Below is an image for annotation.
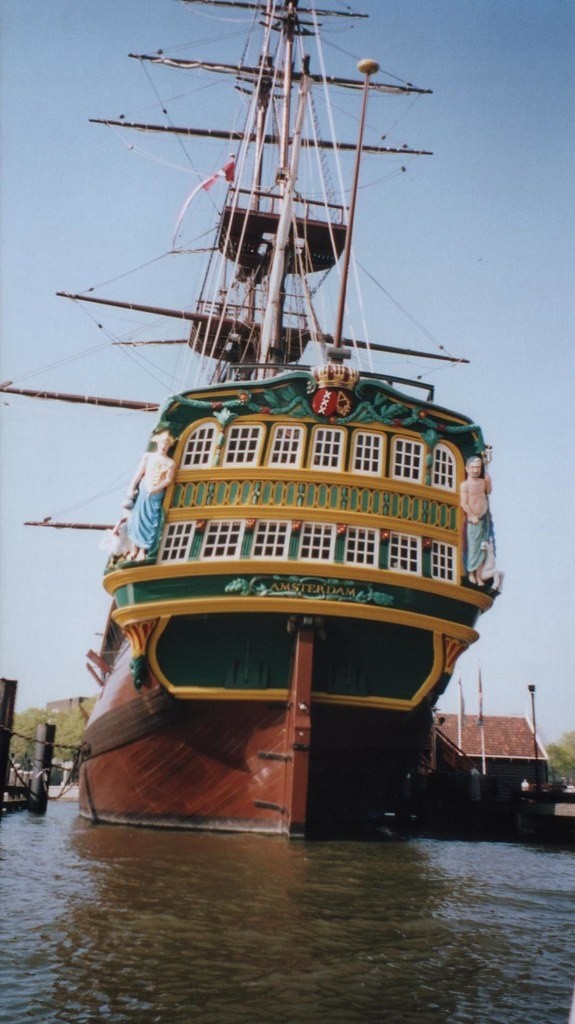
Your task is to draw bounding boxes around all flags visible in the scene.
[474,671,484,726]
[457,678,466,730]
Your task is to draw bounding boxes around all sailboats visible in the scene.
[0,0,504,840]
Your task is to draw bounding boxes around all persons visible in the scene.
[460,454,493,584]
[123,428,176,564]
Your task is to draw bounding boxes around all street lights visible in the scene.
[527,684,542,792]
